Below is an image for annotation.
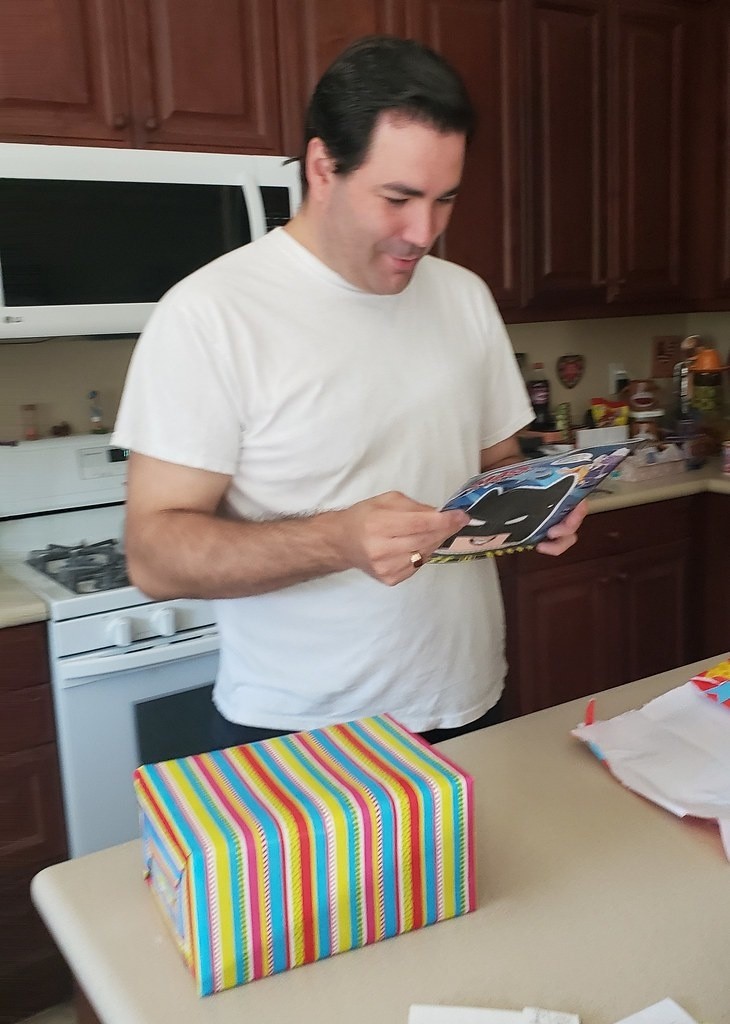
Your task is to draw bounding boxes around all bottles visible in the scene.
[527,363,549,432]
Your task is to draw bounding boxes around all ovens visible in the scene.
[52,626,224,856]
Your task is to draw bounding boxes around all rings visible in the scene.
[410,552,422,567]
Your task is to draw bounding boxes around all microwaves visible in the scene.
[1,143,302,339]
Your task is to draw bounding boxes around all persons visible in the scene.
[108,36,589,746]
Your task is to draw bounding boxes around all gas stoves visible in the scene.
[0,429,217,662]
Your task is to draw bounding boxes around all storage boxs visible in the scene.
[127,710,477,994]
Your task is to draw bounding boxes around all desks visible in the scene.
[28,649,730,1024]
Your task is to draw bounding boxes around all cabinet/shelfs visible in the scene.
[0,0,730,325]
[0,566,82,1024]
[494,458,730,722]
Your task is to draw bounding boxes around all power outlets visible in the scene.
[609,363,625,393]
